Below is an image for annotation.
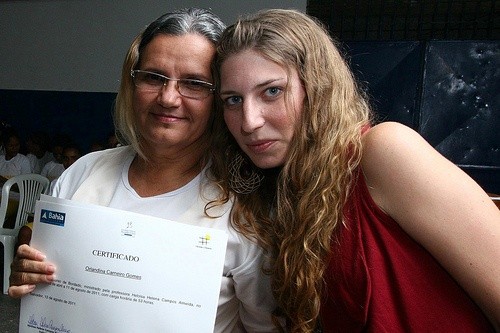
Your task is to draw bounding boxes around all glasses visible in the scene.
[130,68,216,98]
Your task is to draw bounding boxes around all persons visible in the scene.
[0,121,121,188]
[6,8,283,333]
[196,6,500,333]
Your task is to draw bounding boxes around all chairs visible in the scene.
[0,174,54,295]
